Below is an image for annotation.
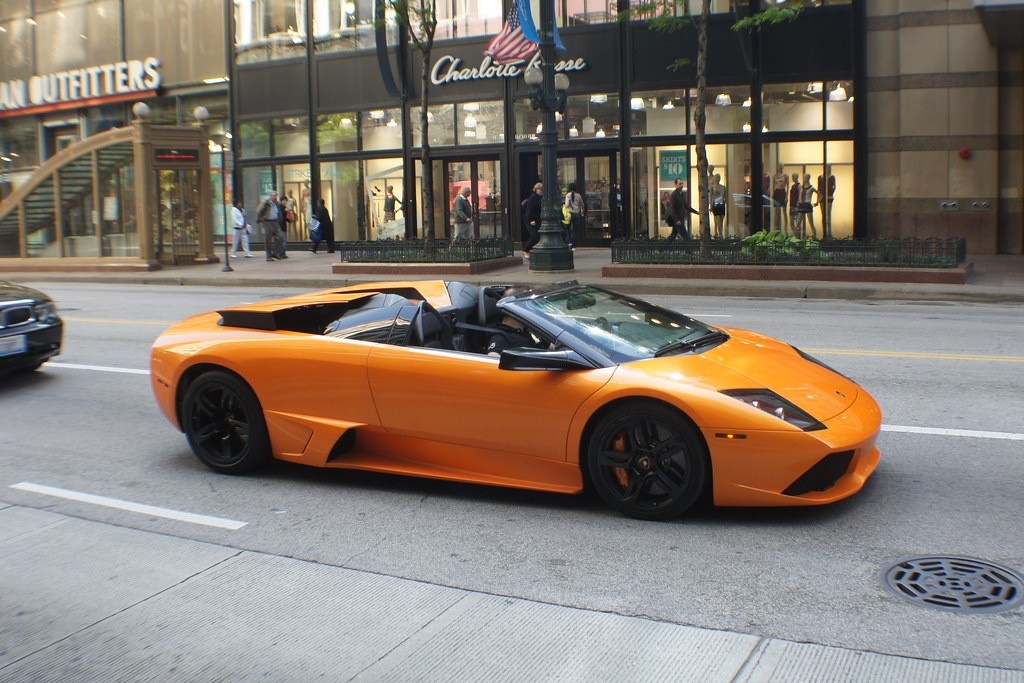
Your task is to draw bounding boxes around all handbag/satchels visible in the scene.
[561,205,571,224]
[246,222,252,233]
[308,217,320,233]
[665,207,673,226]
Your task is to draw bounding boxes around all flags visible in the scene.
[482,0,541,67]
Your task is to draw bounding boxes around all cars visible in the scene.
[0,280,63,378]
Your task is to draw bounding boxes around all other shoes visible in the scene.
[244,253,252,257]
[230,254,236,258]
[568,242,576,251]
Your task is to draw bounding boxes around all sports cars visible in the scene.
[149,278,888,524]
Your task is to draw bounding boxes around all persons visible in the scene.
[789,172,803,236]
[229,197,254,259]
[661,192,670,221]
[449,187,477,256]
[285,189,298,241]
[522,183,544,258]
[308,199,336,253]
[256,192,290,262]
[564,183,585,251]
[300,180,312,241]
[818,162,836,238]
[486,283,556,359]
[706,165,728,241]
[382,185,402,242]
[666,178,700,241]
[772,162,789,238]
[495,192,501,211]
[795,172,823,240]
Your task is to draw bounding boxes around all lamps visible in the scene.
[582,95,596,135]
[0,141,21,162]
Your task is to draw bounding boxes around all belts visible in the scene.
[265,219,276,221]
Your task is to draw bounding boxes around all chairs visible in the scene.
[478,286,503,328]
[407,300,466,352]
